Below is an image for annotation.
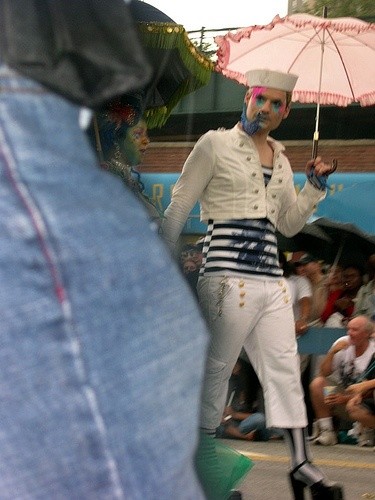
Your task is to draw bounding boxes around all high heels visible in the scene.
[288,459,343,500]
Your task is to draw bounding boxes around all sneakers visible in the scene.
[314,431,338,445]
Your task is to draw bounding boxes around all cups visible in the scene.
[323,386,337,401]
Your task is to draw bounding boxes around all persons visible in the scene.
[177,245,375,445]
[159,69,343,500]
[96,105,161,227]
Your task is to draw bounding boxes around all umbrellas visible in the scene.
[274,223,333,252]
[212,6,375,177]
[311,217,375,261]
[122,0,212,129]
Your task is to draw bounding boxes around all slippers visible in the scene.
[251,426,269,442]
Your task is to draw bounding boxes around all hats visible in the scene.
[245,69,299,92]
